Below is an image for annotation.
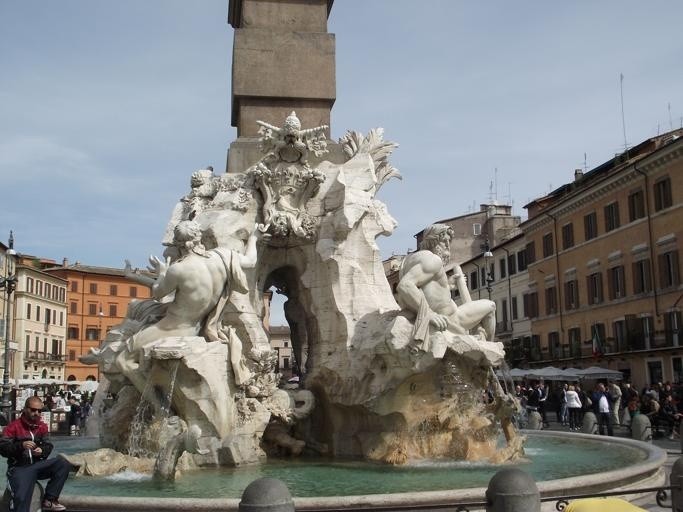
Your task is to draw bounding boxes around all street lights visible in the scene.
[96,304,104,383]
[483,237,492,300]
[0,228,18,427]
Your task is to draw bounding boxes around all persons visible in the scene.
[0,370,99,447]
[393,223,498,344]
[77,219,271,423]
[496,371,683,447]
[0,394,71,511]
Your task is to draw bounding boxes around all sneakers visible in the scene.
[42,499,67,511]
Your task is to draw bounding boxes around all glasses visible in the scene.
[27,407,42,413]
